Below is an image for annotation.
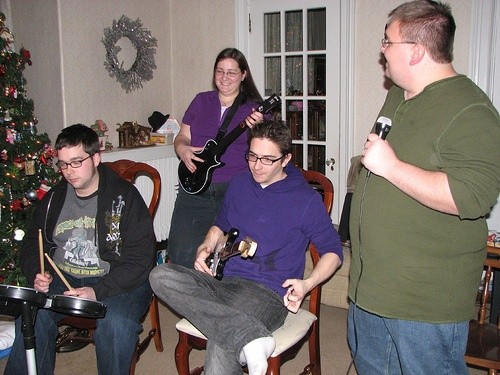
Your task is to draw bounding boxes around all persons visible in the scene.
[3,123,158,375]
[347,0,500,375]
[166,48,266,267]
[147,119,344,375]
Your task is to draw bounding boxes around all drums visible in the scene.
[0,284,47,310]
[49,294,107,319]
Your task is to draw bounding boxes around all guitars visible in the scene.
[177,93,281,195]
[205,228,258,281]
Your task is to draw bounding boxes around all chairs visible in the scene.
[172,170,334,375]
[40,160,163,375]
[463,256,500,375]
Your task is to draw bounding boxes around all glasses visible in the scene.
[56,156,90,169]
[245,150,282,165]
[380,37,414,48]
[215,68,242,77]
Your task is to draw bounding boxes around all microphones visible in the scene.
[367,116,392,177]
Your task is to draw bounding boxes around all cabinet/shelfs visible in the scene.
[100,142,180,265]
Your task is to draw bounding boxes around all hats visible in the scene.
[148,110,170,134]
